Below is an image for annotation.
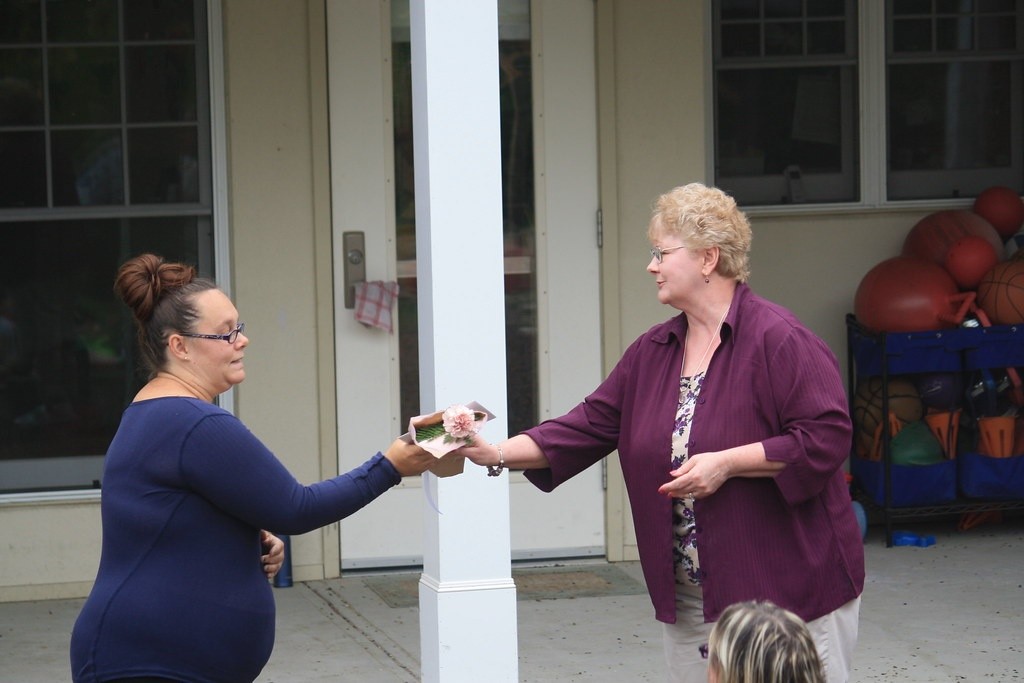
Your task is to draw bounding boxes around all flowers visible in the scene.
[408,405,487,478]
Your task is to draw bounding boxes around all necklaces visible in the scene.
[677,297,730,404]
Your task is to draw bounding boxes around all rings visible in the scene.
[688,491,697,504]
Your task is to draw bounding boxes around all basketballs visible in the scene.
[854,376,922,439]
[978,260,1024,325]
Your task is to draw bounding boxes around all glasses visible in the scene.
[696,643,709,659]
[649,246,687,263]
[165,322,246,344]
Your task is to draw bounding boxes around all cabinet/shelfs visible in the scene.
[846,313,1024,549]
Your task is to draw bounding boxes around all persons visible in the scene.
[68,253,444,683]
[705,596,829,683]
[453,182,866,683]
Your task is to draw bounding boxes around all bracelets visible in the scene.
[486,438,508,480]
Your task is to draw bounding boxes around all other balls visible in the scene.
[946,236,997,287]
[1004,230,1024,260]
[888,421,944,465]
[917,372,975,411]
[974,186,1024,236]
[852,256,959,330]
[903,210,1005,290]
